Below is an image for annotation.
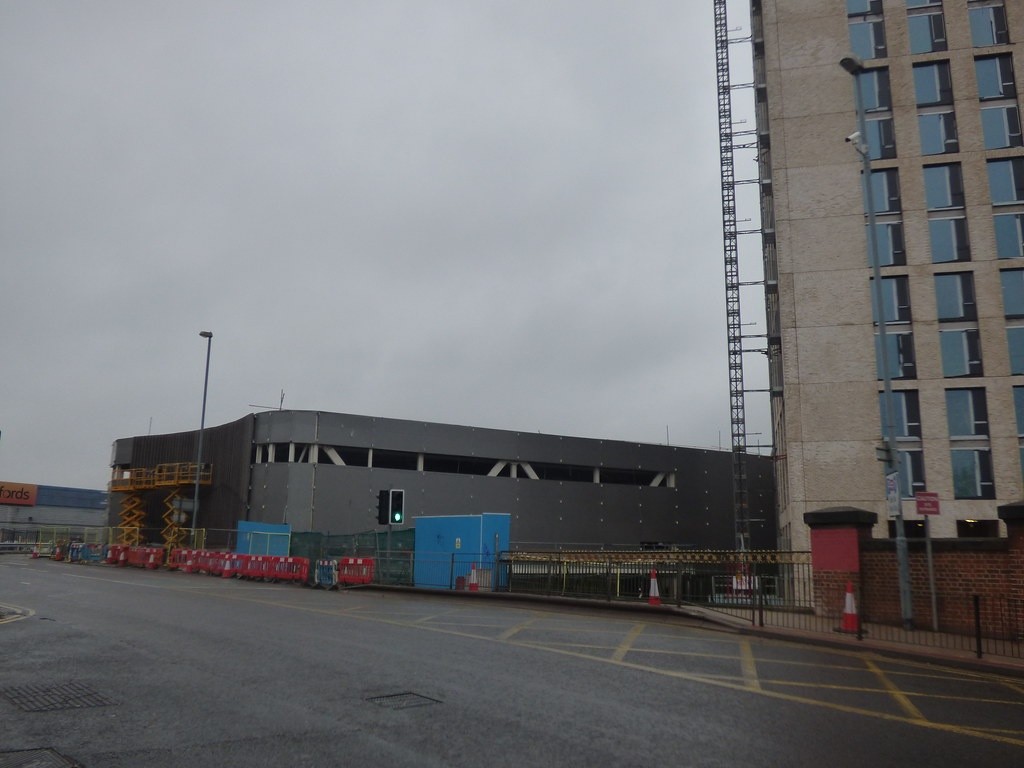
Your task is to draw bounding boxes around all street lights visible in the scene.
[189,331,213,545]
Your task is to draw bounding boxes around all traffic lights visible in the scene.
[375,491,389,524]
[388,488,404,525]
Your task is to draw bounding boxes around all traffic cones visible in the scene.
[186,555,193,573]
[222,557,231,576]
[829,578,870,634]
[468,563,479,593]
[118,551,128,568]
[106,548,114,565]
[146,549,157,570]
[55,546,62,562]
[648,568,662,605]
[29,545,39,559]
